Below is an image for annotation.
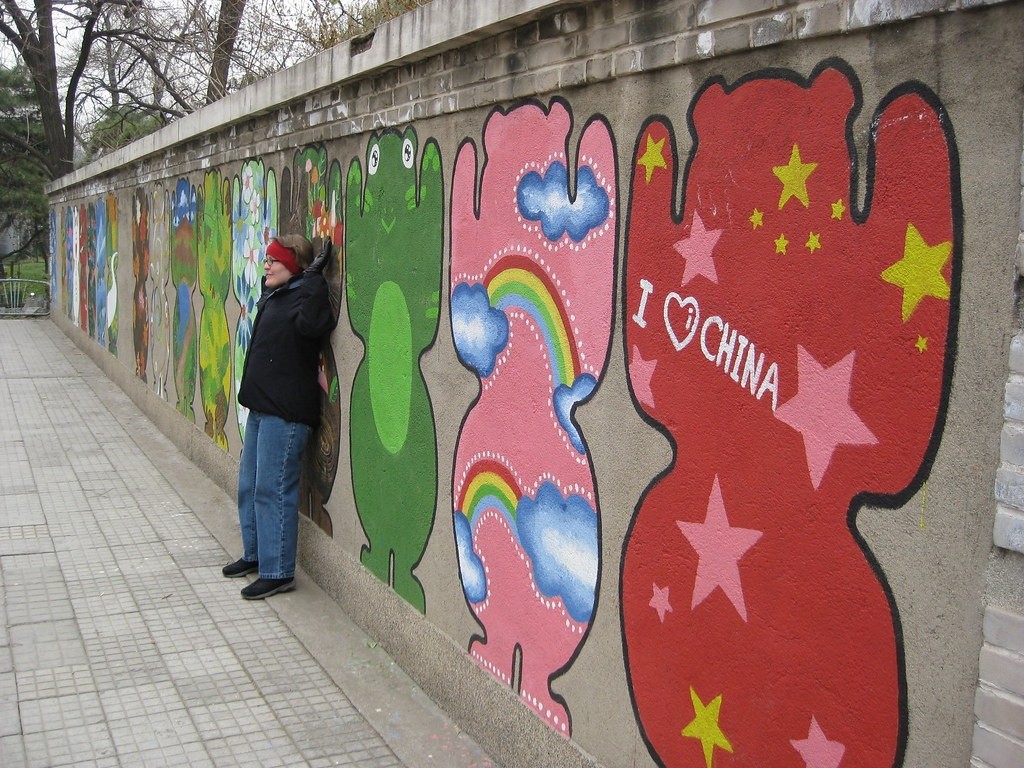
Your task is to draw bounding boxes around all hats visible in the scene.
[266,240,302,274]
[270,235,314,268]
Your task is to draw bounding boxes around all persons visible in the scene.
[222,234,332,600]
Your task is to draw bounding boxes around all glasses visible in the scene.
[263,257,280,265]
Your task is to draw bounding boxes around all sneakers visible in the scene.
[241,576,296,600]
[222,557,258,577]
